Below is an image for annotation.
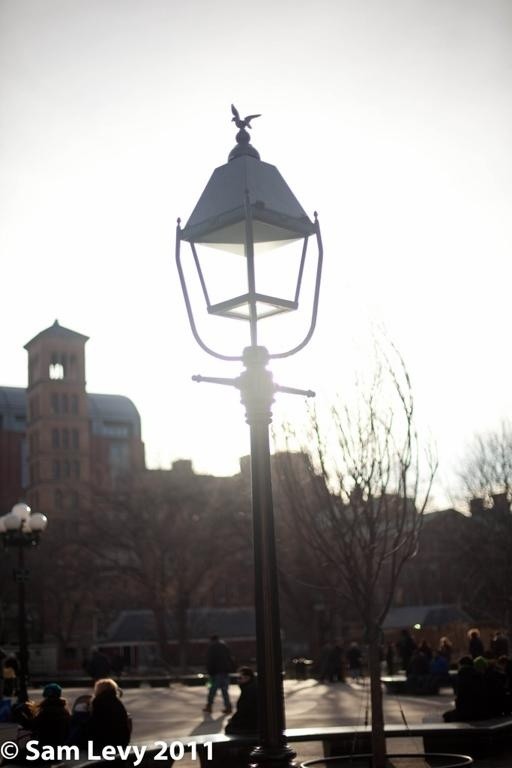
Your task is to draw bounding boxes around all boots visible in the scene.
[203,703,211,712]
[223,705,231,713]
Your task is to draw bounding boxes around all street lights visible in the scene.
[176,104,323,768]
[0,501,48,672]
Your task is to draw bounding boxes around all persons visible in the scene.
[0,646,132,765]
[320,629,512,722]
[226,666,258,734]
[204,634,236,713]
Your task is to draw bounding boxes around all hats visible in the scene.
[42,683,62,697]
